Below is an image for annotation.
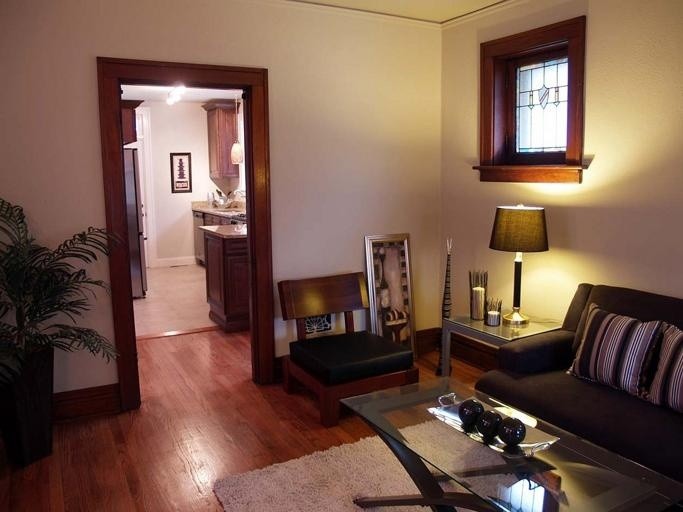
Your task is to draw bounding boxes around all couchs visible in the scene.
[473,283,682,483]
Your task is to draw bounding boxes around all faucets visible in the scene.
[233,189,245,197]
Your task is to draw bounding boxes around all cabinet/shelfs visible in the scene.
[200,98,239,179]
[192,206,250,332]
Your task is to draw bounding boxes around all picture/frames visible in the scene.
[170,152,192,193]
[363,232,417,361]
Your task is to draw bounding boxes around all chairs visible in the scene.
[276,271,419,428]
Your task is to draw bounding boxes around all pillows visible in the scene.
[564,302,683,409]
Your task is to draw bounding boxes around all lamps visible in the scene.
[487,205,549,327]
[230,98,242,165]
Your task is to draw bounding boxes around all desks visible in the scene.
[440,313,562,390]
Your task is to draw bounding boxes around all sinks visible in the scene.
[215,208,243,213]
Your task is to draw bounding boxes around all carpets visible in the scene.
[211,420,518,512]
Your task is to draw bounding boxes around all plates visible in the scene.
[426,392,559,458]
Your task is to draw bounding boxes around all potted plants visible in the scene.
[0,198,125,471]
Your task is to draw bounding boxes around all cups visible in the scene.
[207,192,214,209]
[219,197,224,211]
